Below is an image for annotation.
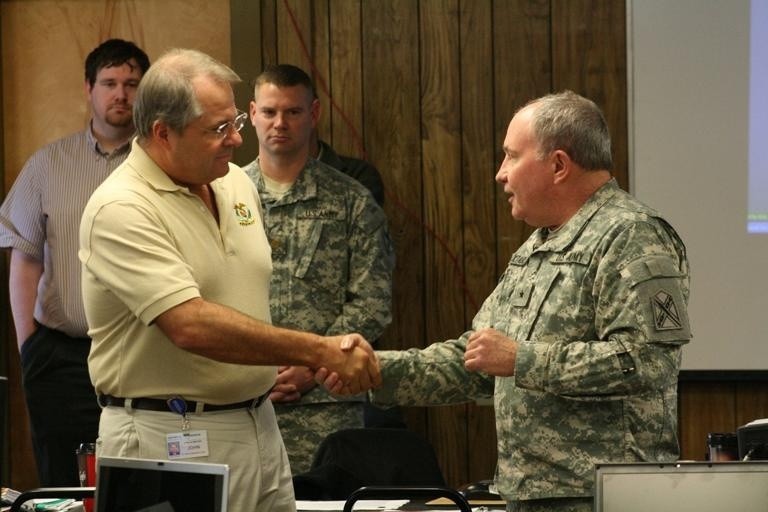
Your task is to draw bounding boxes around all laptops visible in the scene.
[96,456,230,512]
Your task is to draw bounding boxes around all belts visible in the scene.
[101,389,275,413]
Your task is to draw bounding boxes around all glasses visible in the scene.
[155,108,248,139]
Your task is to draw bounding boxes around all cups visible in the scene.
[708,433,740,461]
[76,443,96,512]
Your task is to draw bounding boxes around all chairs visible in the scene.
[292,428,446,499]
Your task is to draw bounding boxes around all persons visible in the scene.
[77,48,384,512]
[312,90,694,512]
[236,63,396,478]
[307,123,384,210]
[0,37,153,489]
